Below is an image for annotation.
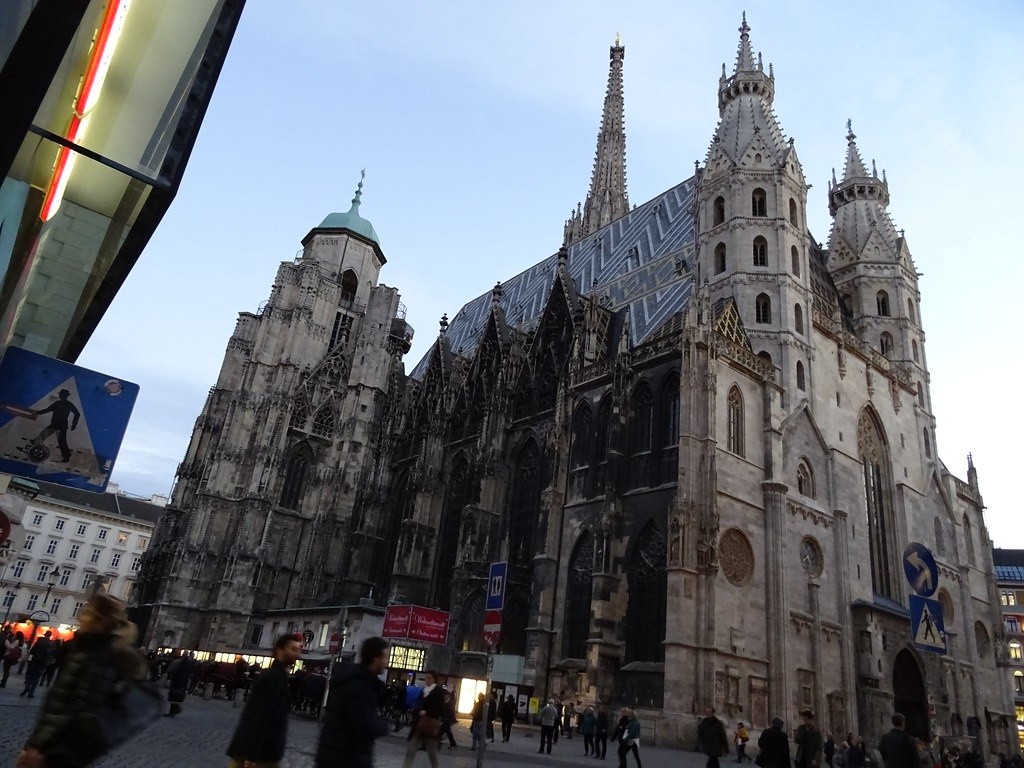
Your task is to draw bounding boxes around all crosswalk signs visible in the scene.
[909,594,948,654]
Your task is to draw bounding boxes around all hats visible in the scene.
[76,595,137,638]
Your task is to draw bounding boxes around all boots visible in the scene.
[163,703,177,718]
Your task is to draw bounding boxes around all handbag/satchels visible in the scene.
[68,673,167,764]
[415,715,443,737]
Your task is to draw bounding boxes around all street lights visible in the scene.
[17,564,61,674]
[1,581,22,632]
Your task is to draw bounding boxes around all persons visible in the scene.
[17,593,163,768]
[578,703,610,760]
[225,634,300,768]
[821,713,1024,768]
[390,670,457,768]
[793,710,823,768]
[755,717,791,768]
[611,707,643,768]
[469,691,518,751]
[733,722,753,764]
[1,625,228,719]
[537,697,575,755]
[696,707,730,768]
[314,637,396,768]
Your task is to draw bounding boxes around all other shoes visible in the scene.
[468,746,477,751]
[590,748,594,756]
[594,755,600,759]
[536,749,543,753]
[489,738,494,744]
[448,744,457,749]
[501,735,509,743]
[600,755,606,761]
[583,752,588,756]
[20,688,34,698]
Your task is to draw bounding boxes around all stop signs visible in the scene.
[483,611,501,649]
[329,633,340,654]
[295,632,304,651]
